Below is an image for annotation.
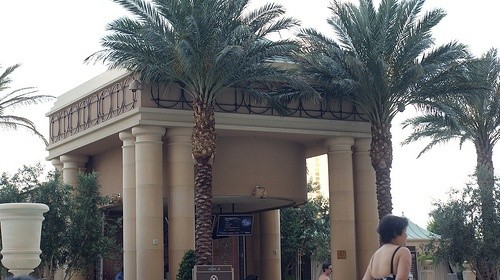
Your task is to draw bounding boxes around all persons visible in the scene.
[361,215,413,280]
[319,263,333,279]
[115,268,123,280]
